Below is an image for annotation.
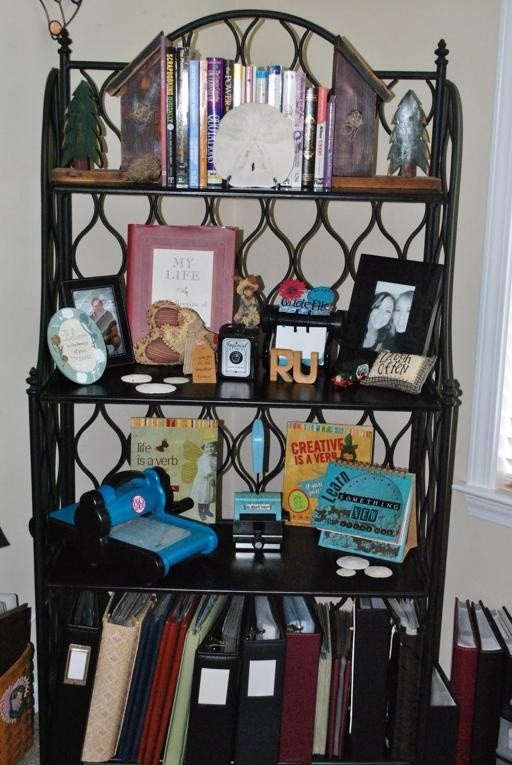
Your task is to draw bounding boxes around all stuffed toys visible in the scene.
[230,273,263,328]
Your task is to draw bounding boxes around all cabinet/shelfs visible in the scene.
[26,3,467,764]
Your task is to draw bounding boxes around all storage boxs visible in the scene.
[1,632,40,765]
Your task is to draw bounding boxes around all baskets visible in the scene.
[0,643,34,765]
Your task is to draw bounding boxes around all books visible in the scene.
[282,419,376,528]
[161,29,337,194]
[1,593,511,765]
[312,458,418,566]
[130,416,220,527]
[127,223,240,366]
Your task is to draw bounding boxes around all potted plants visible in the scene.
[279,278,307,303]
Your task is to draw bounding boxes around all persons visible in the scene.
[358,291,394,353]
[87,298,120,355]
[388,290,413,353]
[190,441,218,521]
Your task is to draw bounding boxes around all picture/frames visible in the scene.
[57,273,133,368]
[128,221,238,344]
[337,248,443,377]
